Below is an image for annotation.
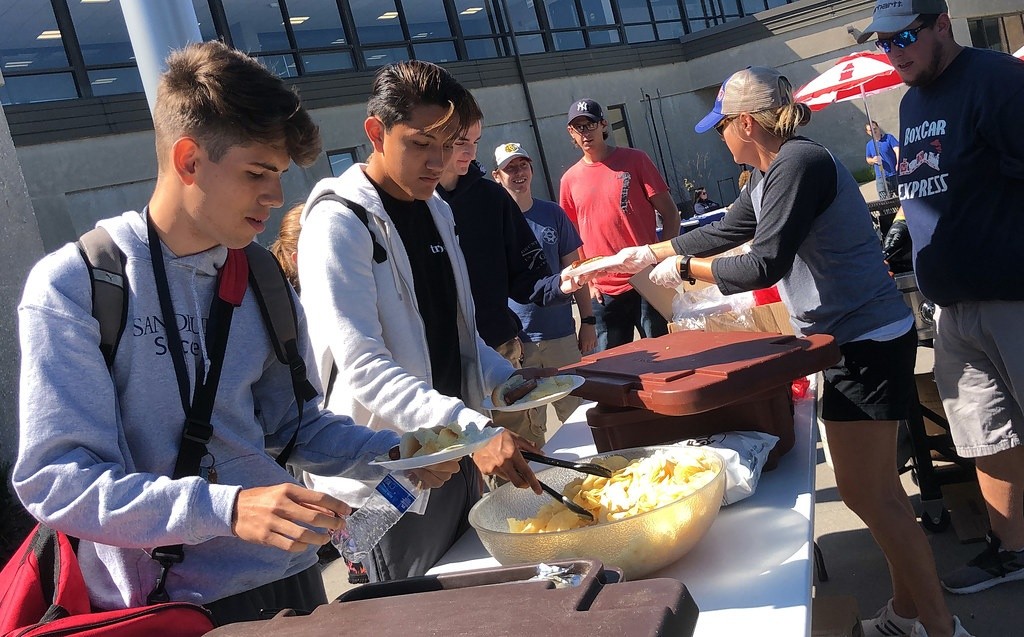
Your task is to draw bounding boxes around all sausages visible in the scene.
[572,255,604,268]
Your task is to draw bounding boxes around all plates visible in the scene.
[368,427,506,472]
[478,374,585,412]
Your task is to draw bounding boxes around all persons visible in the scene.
[865,121,900,199]
[12,40,462,629]
[858,0,1024,594]
[296,61,558,583]
[693,187,720,215]
[272,89,598,370]
[605,65,971,637]
[560,99,681,352]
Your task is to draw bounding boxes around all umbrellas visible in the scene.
[794,49,906,198]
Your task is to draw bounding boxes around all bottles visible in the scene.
[331,471,424,564]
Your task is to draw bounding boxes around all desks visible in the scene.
[656,207,755,258]
[422,373,820,637]
[908,343,977,534]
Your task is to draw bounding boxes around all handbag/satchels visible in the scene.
[0,520,216,637]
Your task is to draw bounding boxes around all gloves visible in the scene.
[884,220,912,259]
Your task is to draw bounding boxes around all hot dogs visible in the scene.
[491,375,539,407]
[375,425,446,461]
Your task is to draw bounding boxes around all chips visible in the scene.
[529,375,574,401]
[412,419,467,456]
[505,448,720,537]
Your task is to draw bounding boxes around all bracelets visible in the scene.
[680,255,695,285]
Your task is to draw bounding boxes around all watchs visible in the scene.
[581,316,596,325]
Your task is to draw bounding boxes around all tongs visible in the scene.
[519,450,612,523]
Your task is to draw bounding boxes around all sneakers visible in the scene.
[941,531,1024,595]
[860,597,972,637]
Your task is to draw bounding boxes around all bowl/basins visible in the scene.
[468,444,727,581]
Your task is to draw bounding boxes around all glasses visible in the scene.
[700,192,708,195]
[714,113,743,137]
[572,122,599,133]
[874,20,929,53]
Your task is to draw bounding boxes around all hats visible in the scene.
[492,142,533,170]
[857,0,948,45]
[694,65,792,135]
[567,99,604,123]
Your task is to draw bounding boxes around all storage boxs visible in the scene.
[197,561,700,637]
[555,330,843,460]
